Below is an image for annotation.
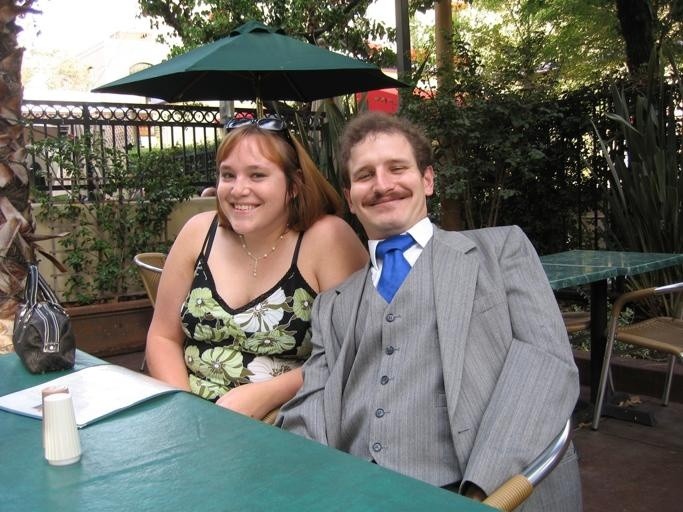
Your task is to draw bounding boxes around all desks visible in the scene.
[0,347,509,512]
[539,249,683,430]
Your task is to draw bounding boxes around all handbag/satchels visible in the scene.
[12,265,75,375]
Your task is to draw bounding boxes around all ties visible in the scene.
[376,232,417,304]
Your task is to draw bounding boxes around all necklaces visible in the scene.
[236,223,290,276]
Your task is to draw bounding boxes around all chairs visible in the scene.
[591,281,683,430]
[562,312,615,397]
[133,252,166,372]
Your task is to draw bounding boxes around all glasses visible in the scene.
[226,118,287,133]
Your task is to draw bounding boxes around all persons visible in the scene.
[142,112,373,447]
[271,108,585,512]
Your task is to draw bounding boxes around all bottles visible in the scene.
[42,385,84,466]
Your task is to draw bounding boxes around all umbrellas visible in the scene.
[88,18,414,120]
